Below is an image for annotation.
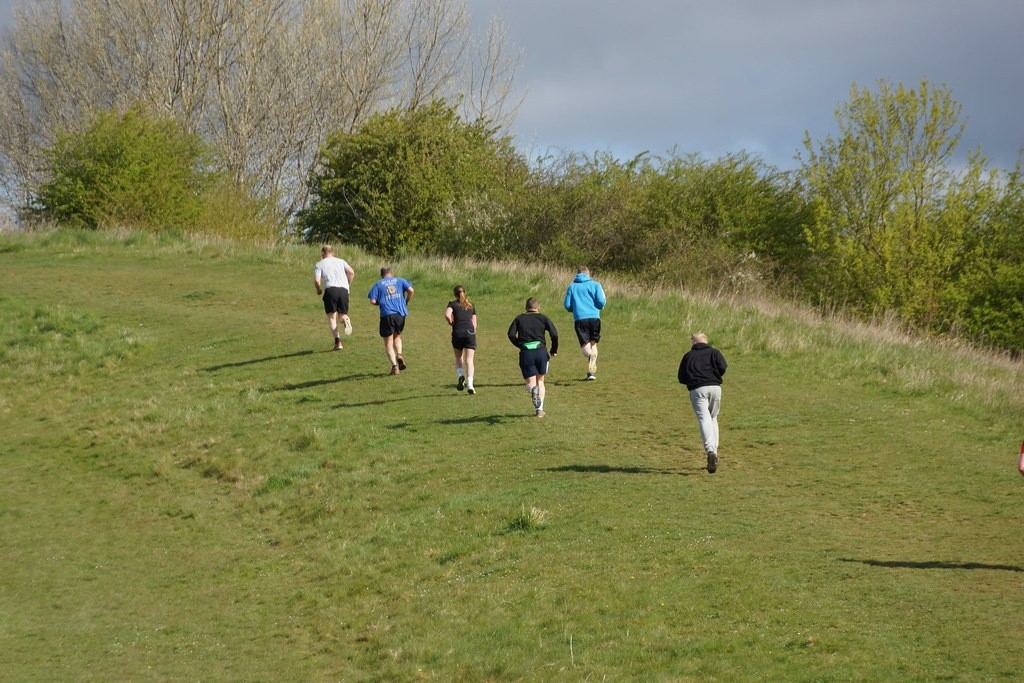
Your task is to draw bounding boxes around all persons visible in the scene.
[444,285,477,394]
[314,245,355,350]
[678,333,728,473]
[564,265,606,380]
[508,297,558,418]
[368,267,414,375]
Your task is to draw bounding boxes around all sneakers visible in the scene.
[586,353,598,380]
[457,375,465,391]
[344,317,353,336]
[707,451,718,473]
[396,355,406,370]
[536,410,545,418]
[333,341,344,350]
[391,366,399,375]
[467,387,476,394]
[531,386,541,408]
